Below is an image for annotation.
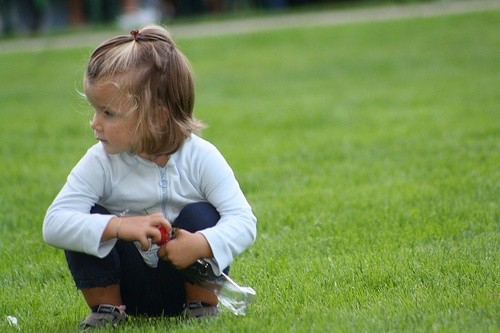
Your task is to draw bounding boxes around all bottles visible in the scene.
[155,226,256,318]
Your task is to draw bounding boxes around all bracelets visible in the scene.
[116,217,121,239]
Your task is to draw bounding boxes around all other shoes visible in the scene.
[186,301,218,317]
[79,304,127,330]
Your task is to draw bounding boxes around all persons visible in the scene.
[41,24,258,331]
